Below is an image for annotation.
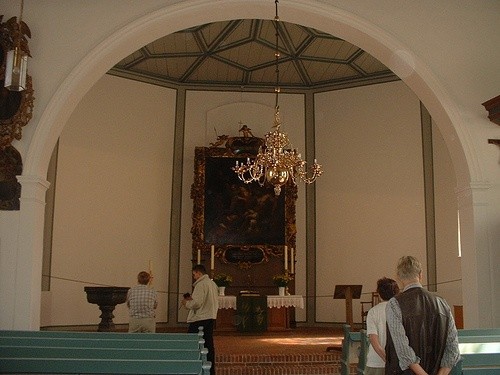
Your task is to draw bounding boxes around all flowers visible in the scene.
[211,272,233,289]
[271,269,295,287]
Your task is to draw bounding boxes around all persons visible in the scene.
[385,255,459,375]
[182,264,219,375]
[126,271,158,333]
[364,277,401,375]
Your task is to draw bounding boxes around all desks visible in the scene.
[213,296,304,337]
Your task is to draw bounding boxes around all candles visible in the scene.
[197,249,201,265]
[210,245,214,270]
[284,245,288,269]
[290,248,295,274]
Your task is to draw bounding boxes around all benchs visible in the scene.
[0,326,212,375]
[340,323,500,375]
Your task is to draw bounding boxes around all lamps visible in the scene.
[3,0,28,92]
[234,0,325,195]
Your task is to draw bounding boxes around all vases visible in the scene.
[219,287,226,297]
[278,287,286,297]
